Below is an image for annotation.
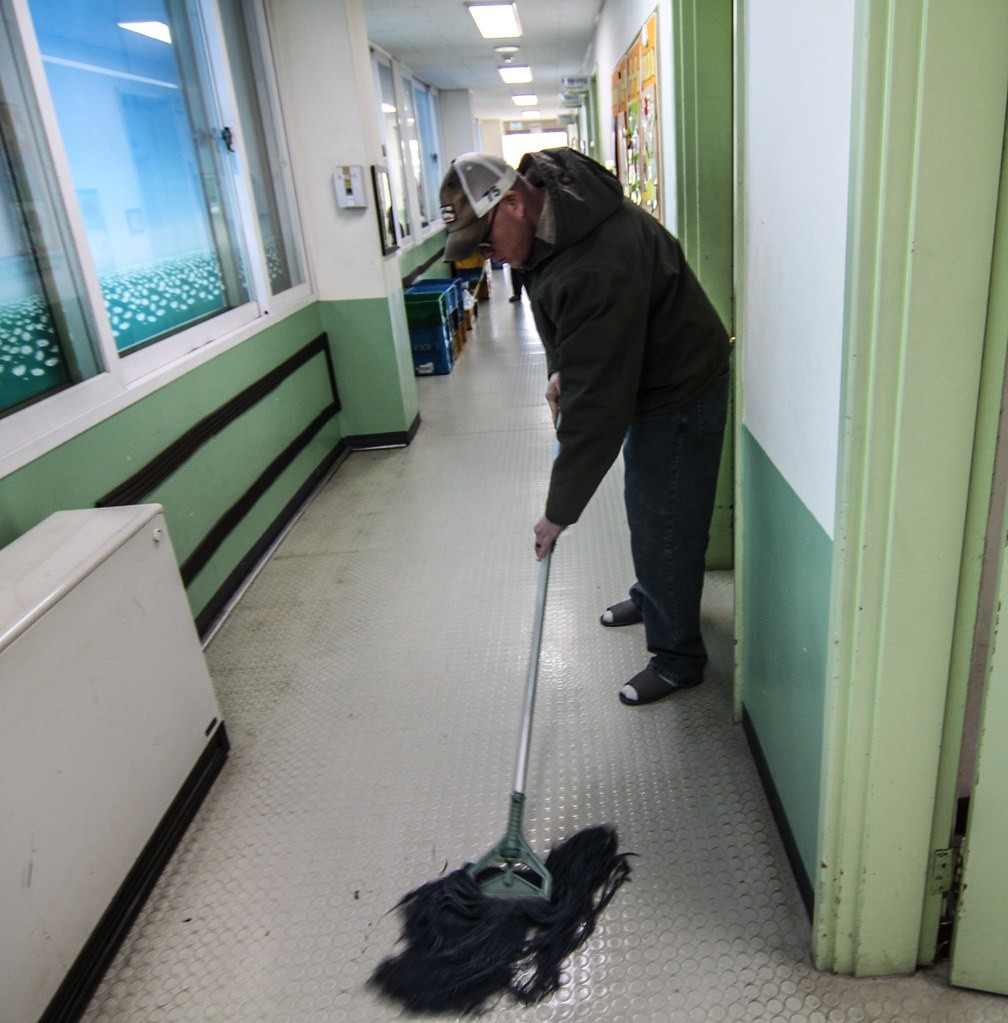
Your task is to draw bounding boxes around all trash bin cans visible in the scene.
[404,278,468,375]
[454,251,492,299]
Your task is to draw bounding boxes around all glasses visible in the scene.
[478,205,499,252]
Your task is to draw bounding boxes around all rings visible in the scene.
[535,542,541,548]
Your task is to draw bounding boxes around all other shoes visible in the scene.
[509,295,520,302]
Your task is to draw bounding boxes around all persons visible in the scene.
[437,144,729,706]
[507,267,529,302]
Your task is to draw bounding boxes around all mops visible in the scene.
[362,393,641,1022]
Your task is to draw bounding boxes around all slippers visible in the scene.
[619,667,704,705]
[600,598,643,627]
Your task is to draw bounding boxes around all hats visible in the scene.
[440,150,517,263]
